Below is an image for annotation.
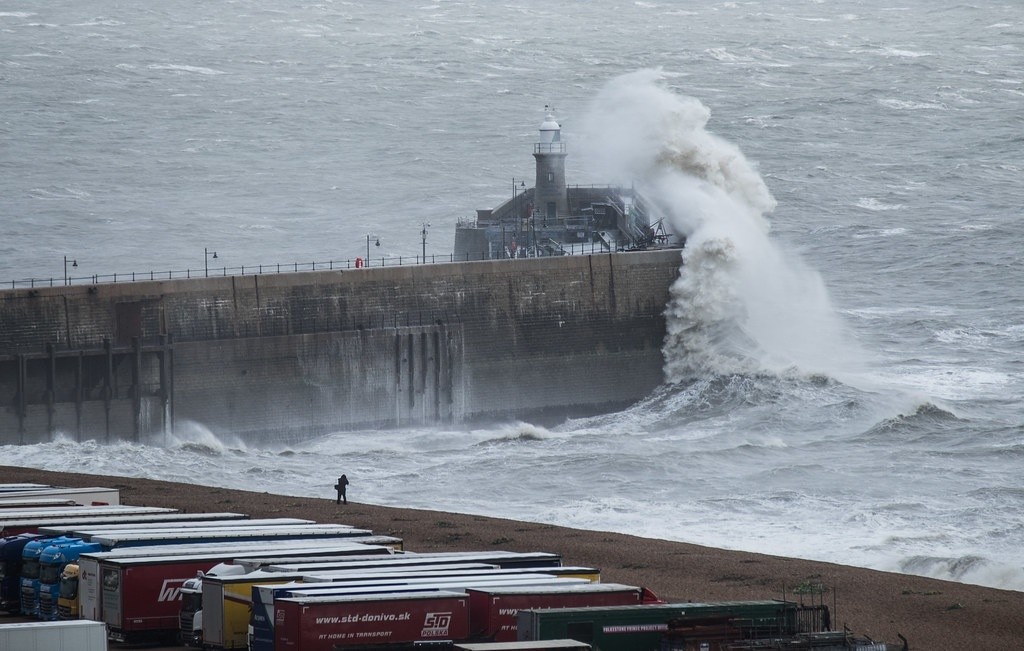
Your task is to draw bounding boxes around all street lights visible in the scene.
[205,248,219,278]
[421,221,431,264]
[64,256,78,287]
[502,178,541,260]
[366,234,380,268]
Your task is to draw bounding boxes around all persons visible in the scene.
[336,474,349,505]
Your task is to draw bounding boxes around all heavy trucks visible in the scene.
[0,480,922,650]
